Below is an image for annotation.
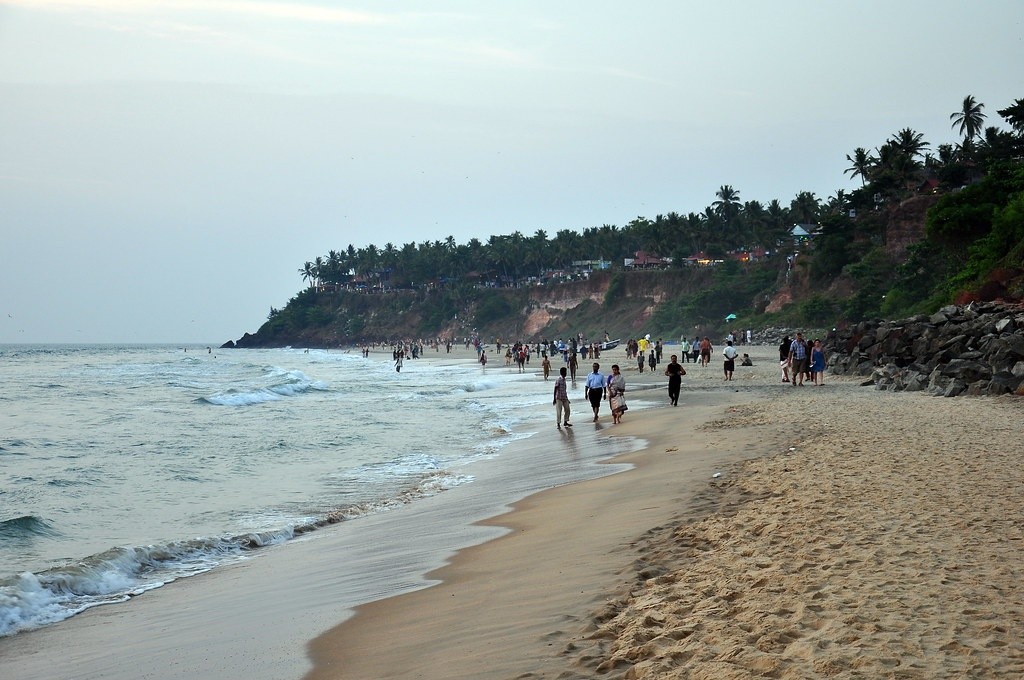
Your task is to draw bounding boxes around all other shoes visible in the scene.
[793,378,796,386]
[805,379,810,381]
[799,383,804,386]
[785,378,790,382]
[782,379,785,382]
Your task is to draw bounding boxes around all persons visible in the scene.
[353,331,826,429]
[664,355,686,406]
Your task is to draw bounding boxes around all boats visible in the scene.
[559,338,621,354]
